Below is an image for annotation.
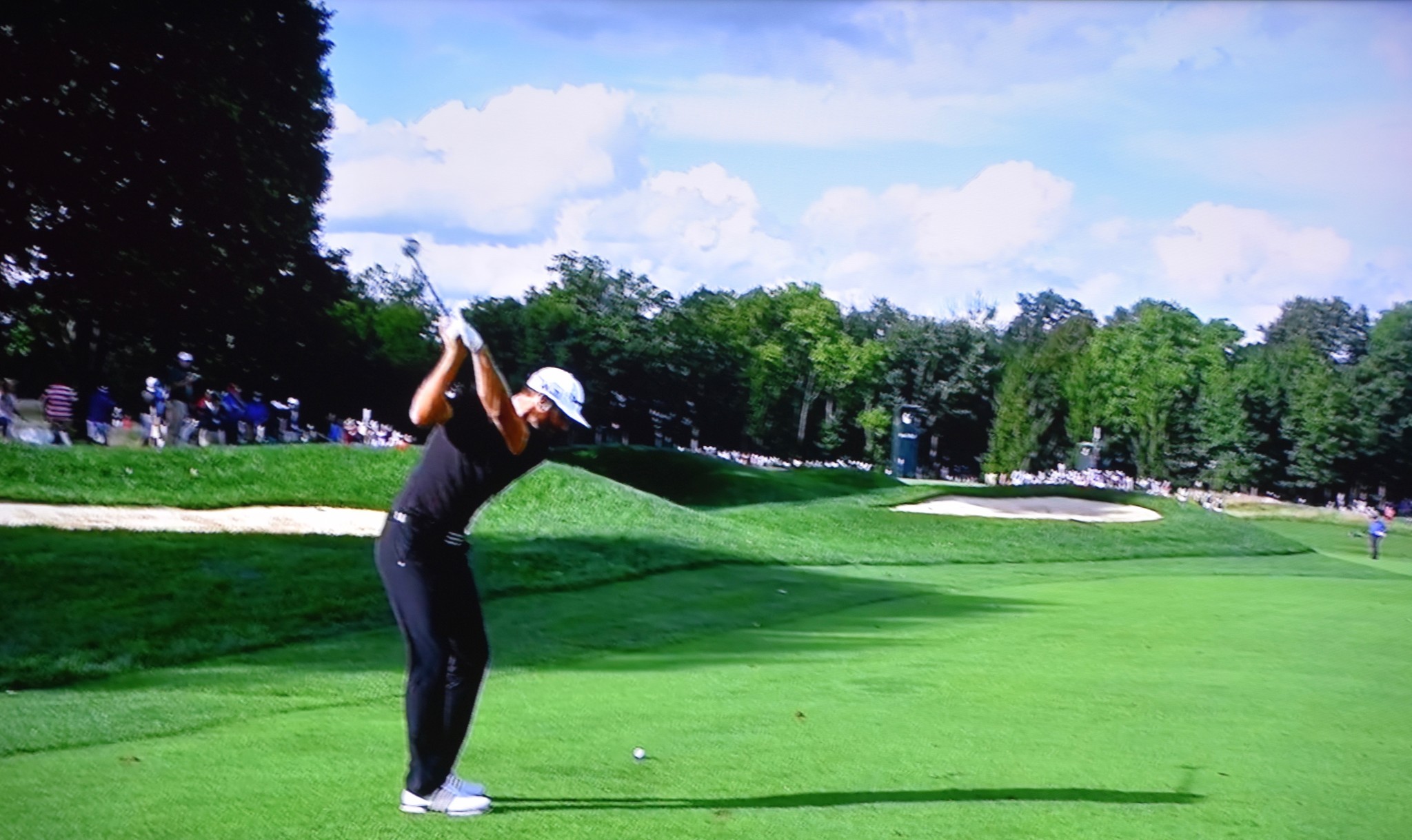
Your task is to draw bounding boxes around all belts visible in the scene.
[392,509,467,546]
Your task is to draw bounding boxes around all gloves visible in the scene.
[444,313,484,352]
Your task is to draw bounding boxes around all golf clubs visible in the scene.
[399,237,450,317]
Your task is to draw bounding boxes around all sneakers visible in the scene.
[442,774,487,796]
[398,780,491,817]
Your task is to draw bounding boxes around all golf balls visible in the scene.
[632,746,646,759]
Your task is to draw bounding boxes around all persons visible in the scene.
[375,308,592,814]
[1368,510,1387,559]
[0,376,410,447]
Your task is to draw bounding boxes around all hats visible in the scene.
[527,366,591,430]
